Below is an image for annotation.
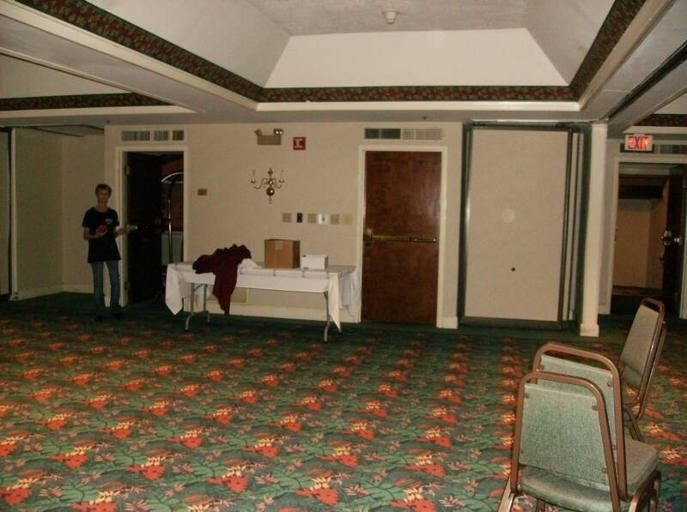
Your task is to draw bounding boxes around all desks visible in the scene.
[168,260,356,344]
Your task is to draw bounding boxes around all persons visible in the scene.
[81,184,128,314]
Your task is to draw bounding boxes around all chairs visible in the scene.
[510,337,663,512]
[606,295,674,442]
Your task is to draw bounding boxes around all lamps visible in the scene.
[248,165,288,204]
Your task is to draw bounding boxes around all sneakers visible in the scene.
[95,316,103,323]
[112,311,123,320]
